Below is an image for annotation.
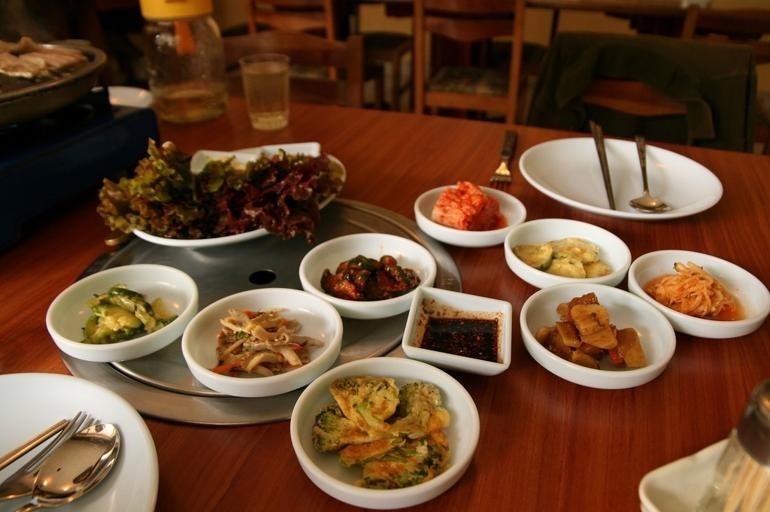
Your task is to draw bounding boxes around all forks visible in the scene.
[487,128,520,192]
[0,408,95,502]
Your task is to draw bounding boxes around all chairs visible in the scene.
[405,1,526,126]
[526,29,762,150]
[236,0,387,109]
[214,31,368,115]
[677,1,770,72]
[345,0,415,113]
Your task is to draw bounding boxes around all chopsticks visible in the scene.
[590,122,616,212]
[0,418,68,473]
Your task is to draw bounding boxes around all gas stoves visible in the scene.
[0,85,161,254]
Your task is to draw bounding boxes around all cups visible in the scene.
[238,51,291,130]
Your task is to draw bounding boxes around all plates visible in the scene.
[299,232,440,321]
[401,285,513,377]
[183,287,344,397]
[503,218,632,290]
[125,153,348,248]
[47,264,199,363]
[519,283,676,390]
[108,82,156,110]
[637,436,728,512]
[287,357,481,511]
[514,135,725,220]
[628,250,770,340]
[0,369,159,512]
[414,184,527,248]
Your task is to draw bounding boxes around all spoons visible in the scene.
[15,414,121,512]
[625,130,667,213]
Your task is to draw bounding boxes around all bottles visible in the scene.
[697,380,770,512]
[139,1,230,124]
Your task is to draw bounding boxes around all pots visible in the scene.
[1,39,109,125]
